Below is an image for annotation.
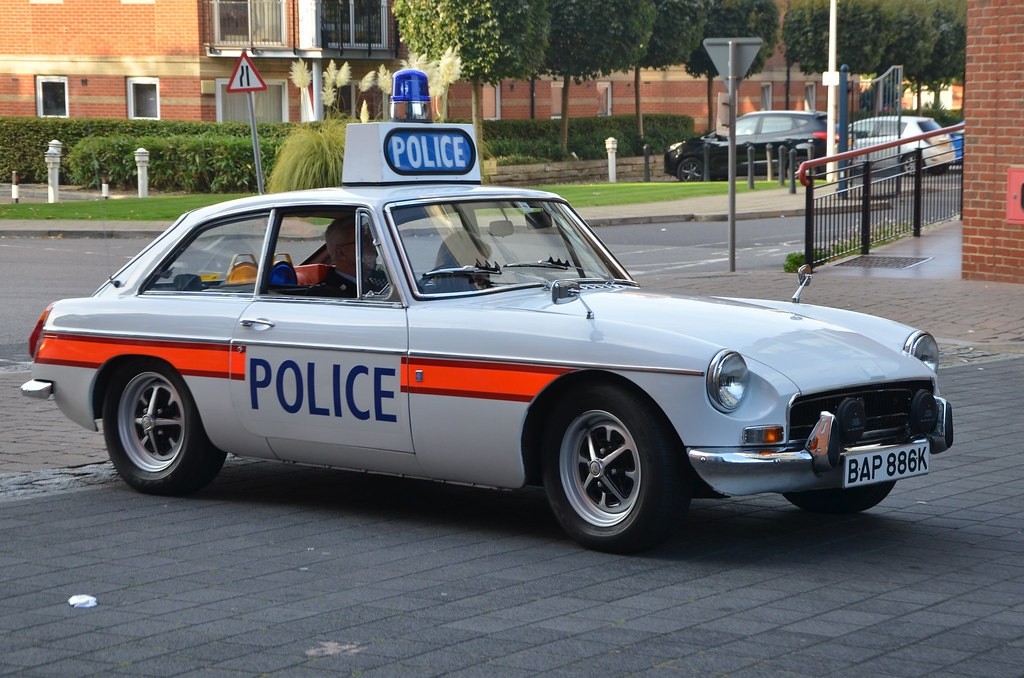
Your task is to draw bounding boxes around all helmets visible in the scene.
[269,260,298,290]
[227,261,259,285]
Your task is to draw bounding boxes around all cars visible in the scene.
[842,116,955,172]
[19,69,954,555]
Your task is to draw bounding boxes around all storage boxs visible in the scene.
[294,264,335,285]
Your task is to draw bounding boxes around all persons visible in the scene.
[308,217,387,299]
[411,233,491,296]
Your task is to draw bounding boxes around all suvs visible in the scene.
[666,109,854,178]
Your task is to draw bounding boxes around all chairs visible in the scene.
[432,231,491,293]
[269,252,299,287]
[228,253,258,283]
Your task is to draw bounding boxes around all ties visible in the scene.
[368,277,388,289]
